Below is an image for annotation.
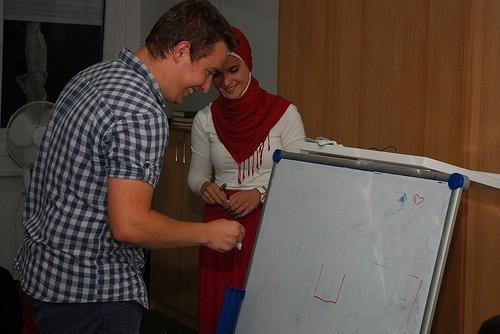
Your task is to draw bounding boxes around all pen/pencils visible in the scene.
[220,183,227,191]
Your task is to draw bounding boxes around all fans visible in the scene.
[4,101,55,194]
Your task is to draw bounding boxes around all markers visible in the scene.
[236,240,245,254]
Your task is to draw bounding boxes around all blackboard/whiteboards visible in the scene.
[231,148,470,334]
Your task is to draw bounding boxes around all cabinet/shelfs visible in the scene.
[278,0,500,334]
[149,128,215,332]
